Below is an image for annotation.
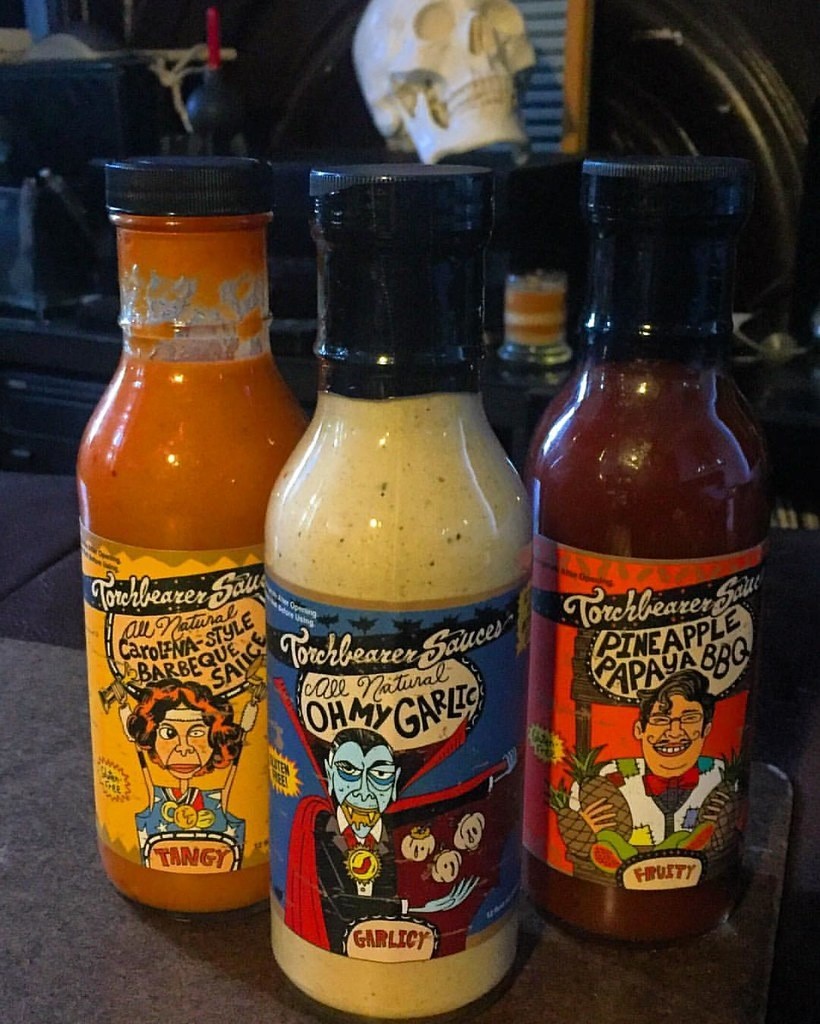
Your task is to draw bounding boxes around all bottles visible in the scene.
[79,155,307,912]
[263,165,533,1020]
[521,162,759,937]
